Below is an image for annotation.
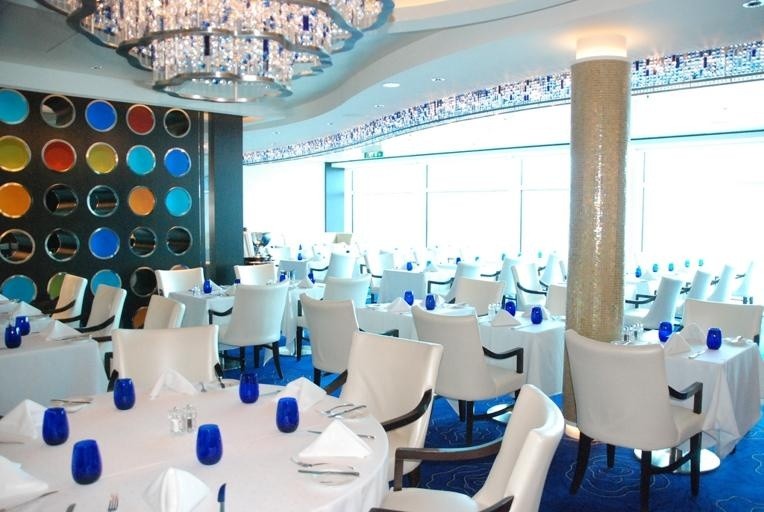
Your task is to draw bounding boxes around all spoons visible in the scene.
[320,402,351,415]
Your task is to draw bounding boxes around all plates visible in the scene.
[327,401,369,419]
[302,463,357,486]
[62,395,90,412]
[210,377,238,388]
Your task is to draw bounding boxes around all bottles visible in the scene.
[487,303,500,324]
[192,284,200,296]
[169,403,196,433]
[622,321,643,342]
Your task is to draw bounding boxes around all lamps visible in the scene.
[31,1,397,113]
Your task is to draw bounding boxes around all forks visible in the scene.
[300,461,353,470]
[105,492,119,511]
[198,380,207,393]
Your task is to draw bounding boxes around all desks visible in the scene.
[2,295,103,412]
[607,329,764,475]
[1,375,391,512]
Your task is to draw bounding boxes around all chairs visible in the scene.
[370,379,566,511]
[409,304,528,449]
[148,230,570,316]
[76,282,129,359]
[624,254,760,327]
[684,297,764,455]
[295,295,401,396]
[207,281,293,381]
[103,296,186,375]
[103,321,225,392]
[38,273,91,328]
[562,325,704,511]
[323,329,446,483]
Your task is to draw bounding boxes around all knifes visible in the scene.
[49,398,91,404]
[65,501,77,512]
[0,490,58,512]
[296,468,359,477]
[689,351,704,359]
[55,334,89,342]
[327,405,366,419]
[217,376,225,390]
[216,482,227,511]
[306,429,376,439]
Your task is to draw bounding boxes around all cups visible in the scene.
[111,377,136,410]
[234,279,239,284]
[3,315,30,348]
[658,321,672,341]
[706,327,722,349]
[280,274,285,282]
[530,306,543,324]
[70,439,103,483]
[237,372,259,404]
[503,300,516,317]
[297,252,302,261]
[41,406,71,447]
[275,397,299,433]
[403,290,414,306]
[193,423,222,465]
[202,279,212,293]
[424,294,436,311]
[633,258,705,279]
[406,262,412,271]
[307,273,315,284]
